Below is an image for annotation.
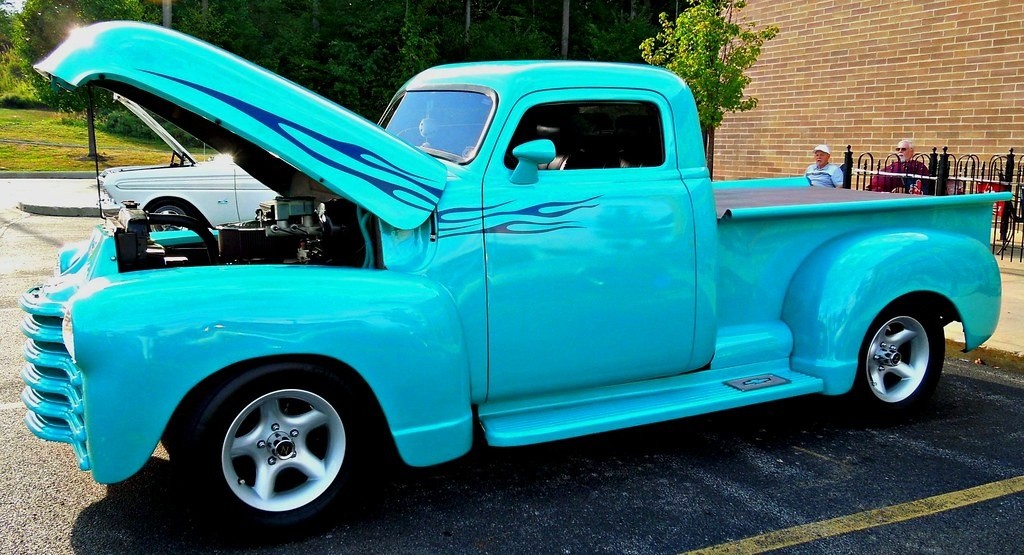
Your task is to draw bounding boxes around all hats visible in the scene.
[814,144,830,154]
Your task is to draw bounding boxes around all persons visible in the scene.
[872,139,928,193]
[805,145,844,189]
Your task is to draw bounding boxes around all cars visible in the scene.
[93,92,281,230]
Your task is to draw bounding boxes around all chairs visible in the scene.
[995,184,1024,255]
[527,105,584,153]
[604,115,655,167]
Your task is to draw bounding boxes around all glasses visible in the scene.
[896,147,910,151]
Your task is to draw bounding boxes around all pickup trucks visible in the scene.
[17,18,1016,542]
